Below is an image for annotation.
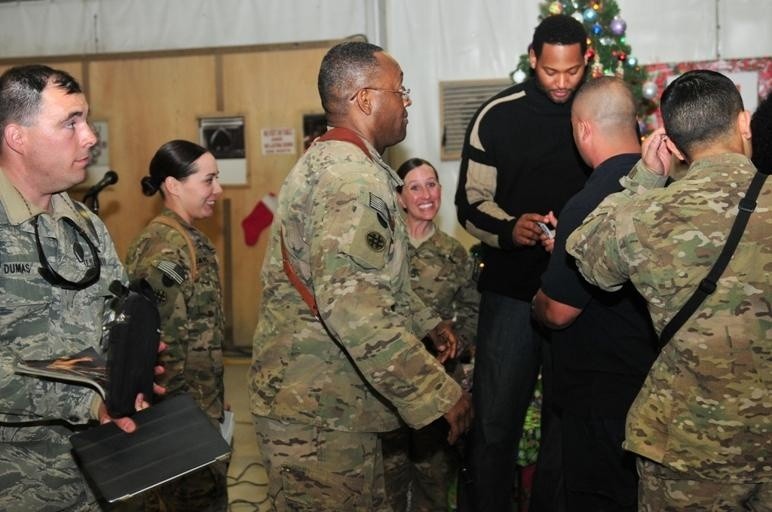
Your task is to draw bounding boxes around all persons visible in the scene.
[248,41,473,512]
[455,13,641,512]
[383,157,480,512]
[534,77,675,512]
[124,139,235,511]
[0,65,167,512]
[565,71,772,512]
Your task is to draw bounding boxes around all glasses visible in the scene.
[30,220,102,290]
[350,88,411,101]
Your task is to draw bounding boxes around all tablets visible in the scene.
[68,392,234,504]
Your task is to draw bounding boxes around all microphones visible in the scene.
[84,170,120,200]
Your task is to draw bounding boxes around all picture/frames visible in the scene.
[298,111,328,156]
[198,116,248,189]
[75,119,110,189]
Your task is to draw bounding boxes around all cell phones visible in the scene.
[538,219,556,239]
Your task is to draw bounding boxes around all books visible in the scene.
[14,347,107,401]
